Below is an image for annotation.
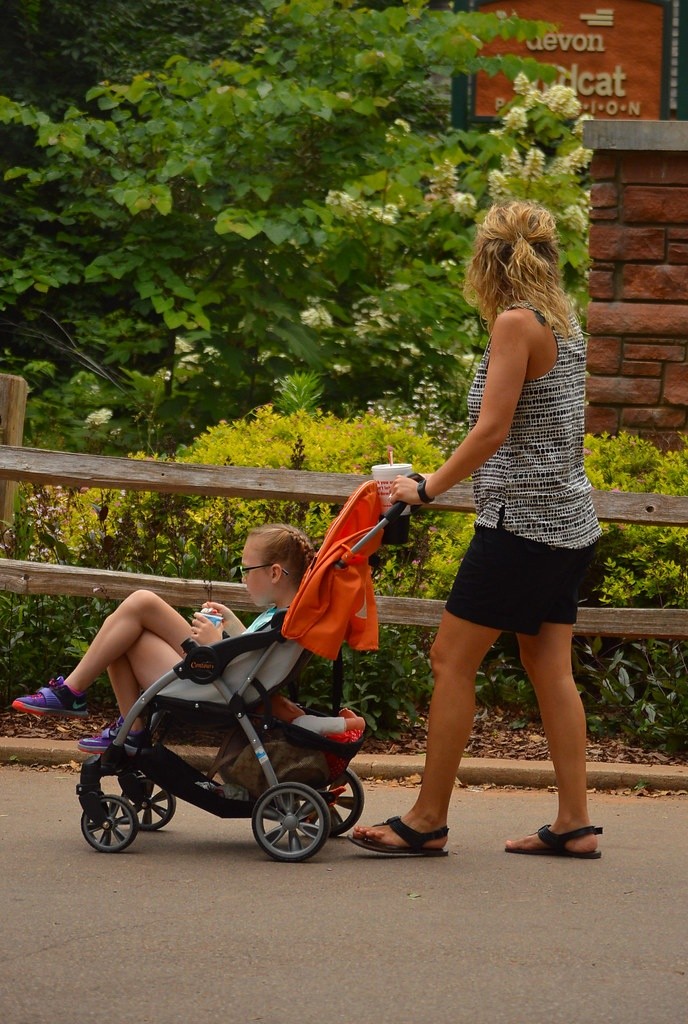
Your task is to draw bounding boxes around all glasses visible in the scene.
[240,563,289,579]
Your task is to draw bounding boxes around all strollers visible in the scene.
[74,472,426,863]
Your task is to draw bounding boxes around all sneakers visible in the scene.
[12,676,88,718]
[77,716,152,756]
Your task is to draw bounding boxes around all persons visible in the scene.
[346,200,605,858]
[258,695,366,735]
[12,523,316,757]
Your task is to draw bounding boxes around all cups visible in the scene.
[372,464,412,515]
[193,612,224,635]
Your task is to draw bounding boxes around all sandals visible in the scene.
[505,824,603,860]
[347,816,449,857]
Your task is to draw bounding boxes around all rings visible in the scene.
[387,494,391,500]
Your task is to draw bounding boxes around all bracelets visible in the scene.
[417,480,434,503]
